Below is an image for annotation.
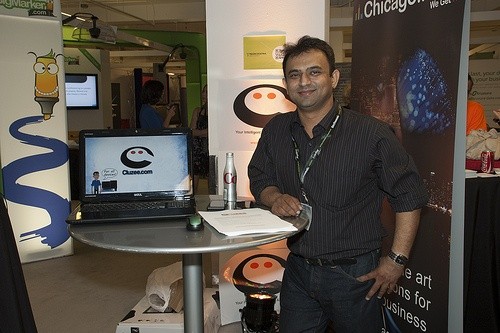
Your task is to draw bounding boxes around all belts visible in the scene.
[303,257,357,267]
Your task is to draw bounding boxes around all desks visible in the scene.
[67,194,309,333]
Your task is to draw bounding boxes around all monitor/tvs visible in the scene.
[65,73,99,110]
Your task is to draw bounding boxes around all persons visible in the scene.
[493,108,500,128]
[466,72,487,135]
[190,85,208,195]
[139,80,177,129]
[248,35,428,333]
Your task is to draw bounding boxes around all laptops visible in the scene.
[65,128,195,224]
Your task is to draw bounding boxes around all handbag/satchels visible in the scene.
[466,128,500,160]
[145,261,206,312]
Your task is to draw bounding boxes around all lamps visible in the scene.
[62,12,101,38]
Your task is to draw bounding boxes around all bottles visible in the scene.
[223,152,237,202]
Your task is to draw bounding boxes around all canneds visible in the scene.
[480,150,494,173]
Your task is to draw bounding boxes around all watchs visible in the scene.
[387,250,409,267]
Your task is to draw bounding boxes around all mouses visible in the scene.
[186,215,203,231]
